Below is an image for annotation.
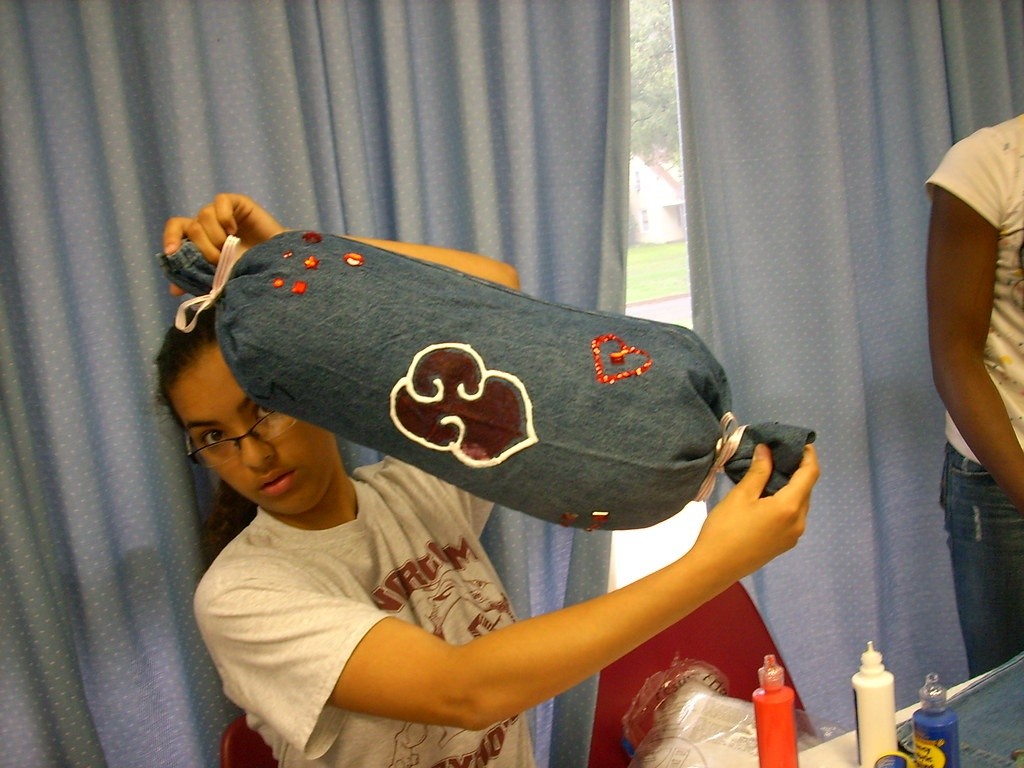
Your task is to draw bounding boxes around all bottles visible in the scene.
[851,640,898,768]
[752,654,799,768]
[911,671,961,768]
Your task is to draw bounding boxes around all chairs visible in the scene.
[589,579,822,767]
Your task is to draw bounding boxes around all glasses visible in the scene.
[185,409,298,469]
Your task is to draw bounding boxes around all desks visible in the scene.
[794,653,1024,767]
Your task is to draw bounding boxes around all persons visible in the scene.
[926,115,1024,674]
[154,194,820,768]
